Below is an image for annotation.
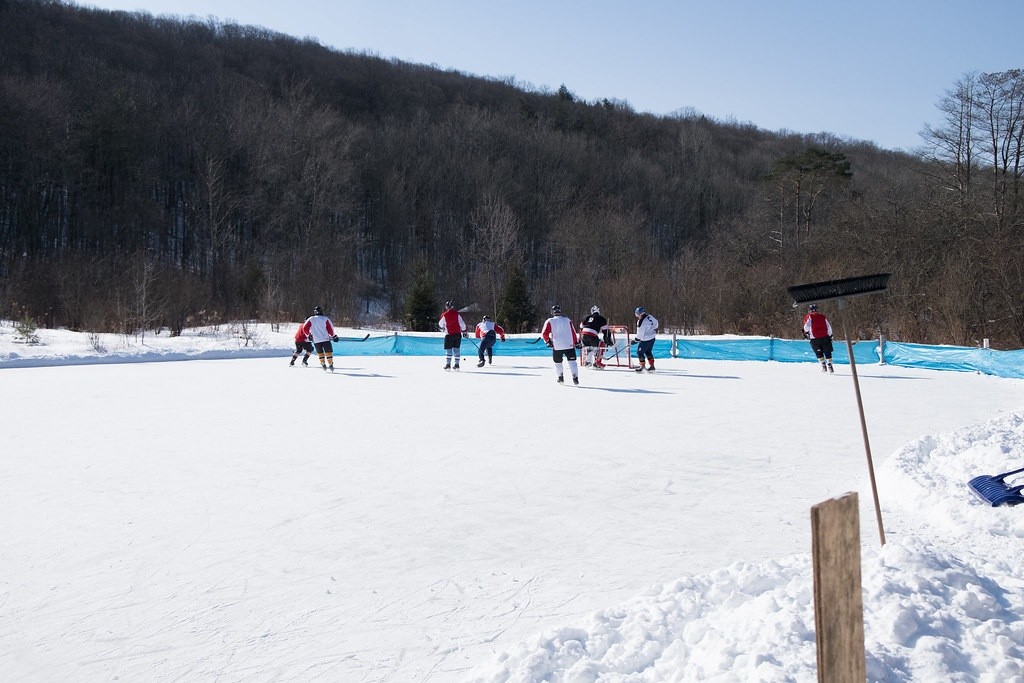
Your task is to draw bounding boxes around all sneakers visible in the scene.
[478,359,485,367]
[573,377,579,386]
[635,365,647,374]
[647,365,655,371]
[489,356,492,364]
[301,354,309,367]
[453,363,460,371]
[443,364,451,372]
[329,362,334,372]
[593,363,605,370]
[584,361,592,368]
[289,355,297,367]
[557,376,564,385]
[322,364,327,371]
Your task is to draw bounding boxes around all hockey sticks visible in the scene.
[583,342,617,350]
[600,343,631,360]
[834,337,860,345]
[469,338,487,355]
[497,336,541,344]
[339,334,370,342]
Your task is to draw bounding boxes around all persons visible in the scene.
[631,306,659,374]
[289,316,314,366]
[438,301,467,370]
[580,305,613,369]
[475,315,505,368]
[541,305,582,384]
[803,304,834,374]
[303,306,339,374]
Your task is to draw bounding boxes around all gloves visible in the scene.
[575,340,582,349]
[332,335,339,343]
[630,340,638,345]
[545,338,554,347]
[500,337,505,342]
[803,331,810,339]
[308,334,313,341]
[463,332,467,337]
[830,335,834,342]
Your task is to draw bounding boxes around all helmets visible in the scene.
[635,306,645,315]
[550,304,562,316]
[445,301,454,309]
[809,304,818,310]
[590,305,600,316]
[313,305,324,315]
[482,315,491,322]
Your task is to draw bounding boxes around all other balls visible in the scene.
[464,358,466,360]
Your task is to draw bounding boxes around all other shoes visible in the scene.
[827,363,833,373]
[822,362,827,372]
